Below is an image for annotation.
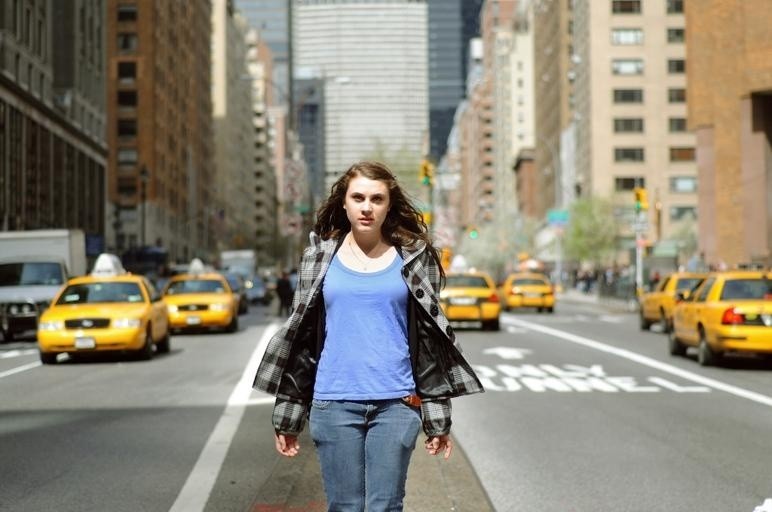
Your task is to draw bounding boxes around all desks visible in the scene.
[237,71,352,268]
[140,162,151,249]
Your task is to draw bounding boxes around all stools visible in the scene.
[398,394,422,409]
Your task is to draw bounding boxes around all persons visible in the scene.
[249,160,484,512]
[569,260,660,301]
[276,267,293,318]
[288,266,300,291]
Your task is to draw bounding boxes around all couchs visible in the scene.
[443,249,452,271]
[421,161,432,186]
[468,226,479,239]
[633,187,649,211]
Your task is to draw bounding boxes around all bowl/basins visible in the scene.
[348,236,383,274]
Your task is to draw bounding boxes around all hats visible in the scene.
[158,256,240,336]
[667,268,772,368]
[34,249,171,363]
[439,254,505,334]
[499,267,555,316]
[634,270,703,332]
[111,242,299,314]
